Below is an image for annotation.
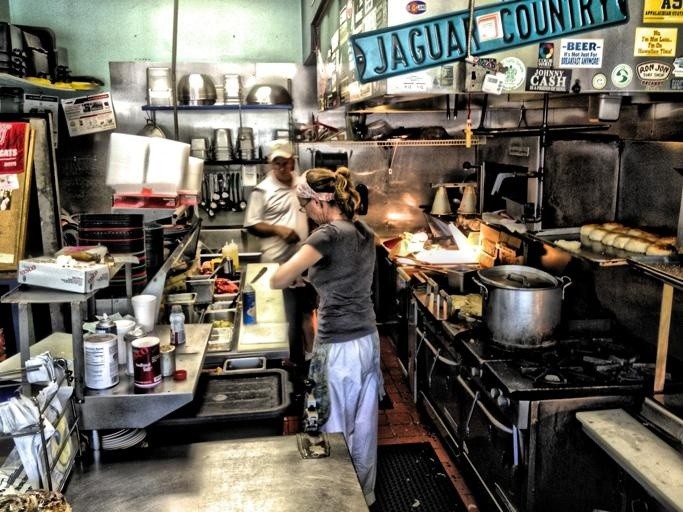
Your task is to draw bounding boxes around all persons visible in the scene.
[243,146,308,266]
[271,165,384,507]
[1,191,11,210]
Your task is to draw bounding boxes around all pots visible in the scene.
[473,264,571,351]
[136,118,166,138]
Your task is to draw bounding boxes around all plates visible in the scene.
[77,212,148,293]
[158,370,290,430]
[539,225,630,266]
[627,252,682,289]
[97,427,147,451]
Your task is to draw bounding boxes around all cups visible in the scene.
[131,294,158,334]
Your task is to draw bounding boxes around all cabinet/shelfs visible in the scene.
[294,132,487,149]
[142,103,294,233]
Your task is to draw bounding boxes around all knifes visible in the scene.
[250,267,267,284]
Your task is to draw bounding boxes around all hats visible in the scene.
[267,138,293,161]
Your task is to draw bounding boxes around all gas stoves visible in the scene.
[457,323,671,407]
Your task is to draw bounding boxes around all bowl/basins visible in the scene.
[184,274,216,305]
[212,129,234,161]
[222,74,243,106]
[177,73,218,106]
[245,83,293,106]
[237,127,256,161]
[163,292,196,324]
[112,319,136,334]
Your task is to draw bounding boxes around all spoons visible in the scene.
[199,172,246,217]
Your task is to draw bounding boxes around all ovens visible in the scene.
[470,129,621,235]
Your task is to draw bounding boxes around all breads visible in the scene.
[646,244,677,256]
[602,232,625,246]
[602,221,624,231]
[580,223,602,236]
[627,228,648,236]
[611,227,631,233]
[624,238,652,254]
[638,232,661,242]
[588,228,610,240]
[655,236,677,246]
[613,236,633,249]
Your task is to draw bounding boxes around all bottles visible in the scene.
[169,304,187,346]
[95,314,118,336]
[123,329,146,377]
[221,241,231,258]
[230,238,240,270]
[242,282,257,325]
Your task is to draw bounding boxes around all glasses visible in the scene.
[273,162,291,169]
[298,199,311,213]
